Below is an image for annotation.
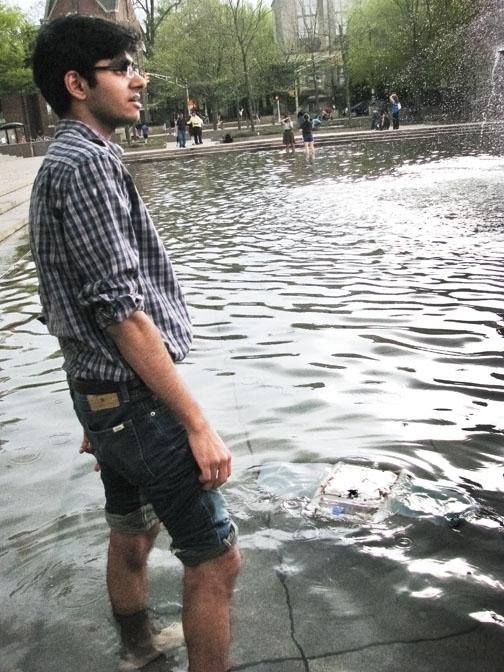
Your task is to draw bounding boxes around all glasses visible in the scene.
[93,63,140,79]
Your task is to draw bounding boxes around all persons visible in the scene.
[279,113,297,156]
[301,113,315,160]
[27,14,246,672]
[130,94,403,148]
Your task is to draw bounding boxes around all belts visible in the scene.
[68,377,143,394]
